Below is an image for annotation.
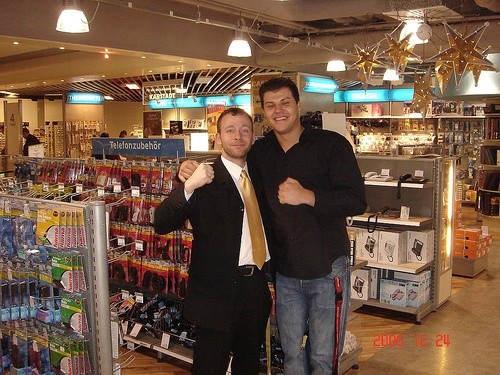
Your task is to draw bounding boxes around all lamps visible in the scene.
[55,0,90,34]
[383,60,399,81]
[227,17,252,57]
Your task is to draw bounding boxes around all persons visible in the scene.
[23,128,40,152]
[177,76,366,375]
[89,130,128,161]
[153,107,278,375]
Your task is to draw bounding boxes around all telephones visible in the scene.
[353,276,364,293]
[364,172,394,182]
[391,288,405,300]
[383,240,395,257]
[408,289,418,301]
[364,236,376,253]
[400,173,429,184]
[411,238,424,256]
[377,206,400,218]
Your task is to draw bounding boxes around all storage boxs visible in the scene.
[351,267,431,308]
[347,225,434,265]
[454,201,493,259]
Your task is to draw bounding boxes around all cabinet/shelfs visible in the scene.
[347,154,456,326]
[478,140,500,240]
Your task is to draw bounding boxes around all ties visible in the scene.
[240,169,267,271]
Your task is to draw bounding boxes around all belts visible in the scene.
[240,266,255,277]
